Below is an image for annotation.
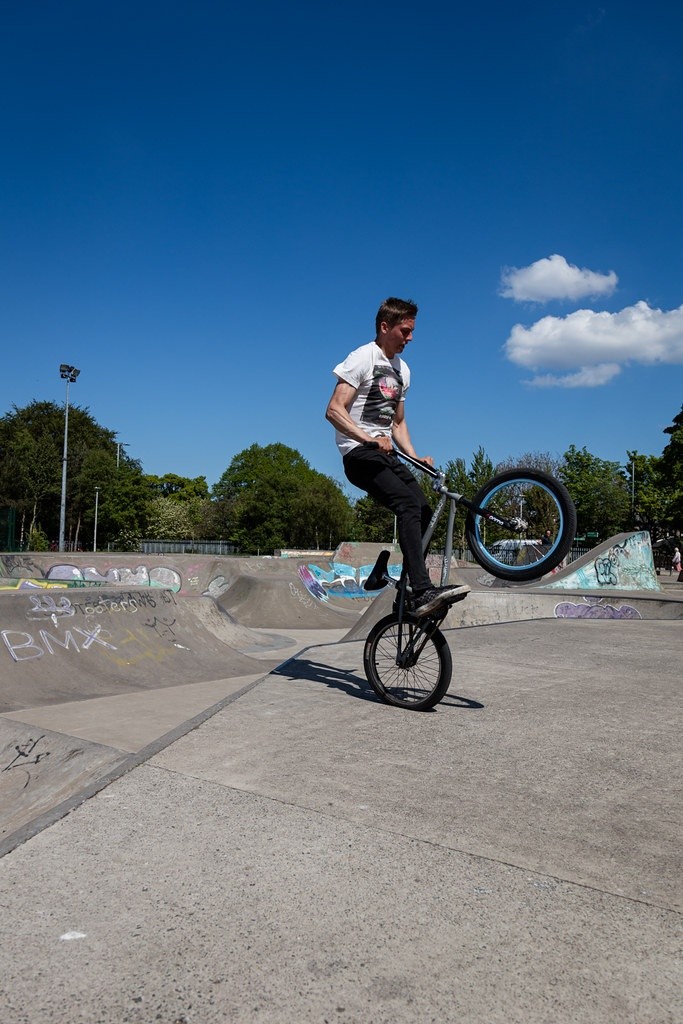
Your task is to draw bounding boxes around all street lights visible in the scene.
[94,487,100,552]
[59,363,81,553]
[117,442,130,468]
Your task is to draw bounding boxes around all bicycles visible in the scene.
[363,441,577,711]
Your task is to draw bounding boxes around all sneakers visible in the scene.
[413,584,471,618]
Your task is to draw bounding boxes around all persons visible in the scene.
[326,297,471,617]
[672,547,681,571]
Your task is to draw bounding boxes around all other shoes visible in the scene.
[391,598,421,614]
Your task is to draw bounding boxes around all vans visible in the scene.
[487,539,542,561]
